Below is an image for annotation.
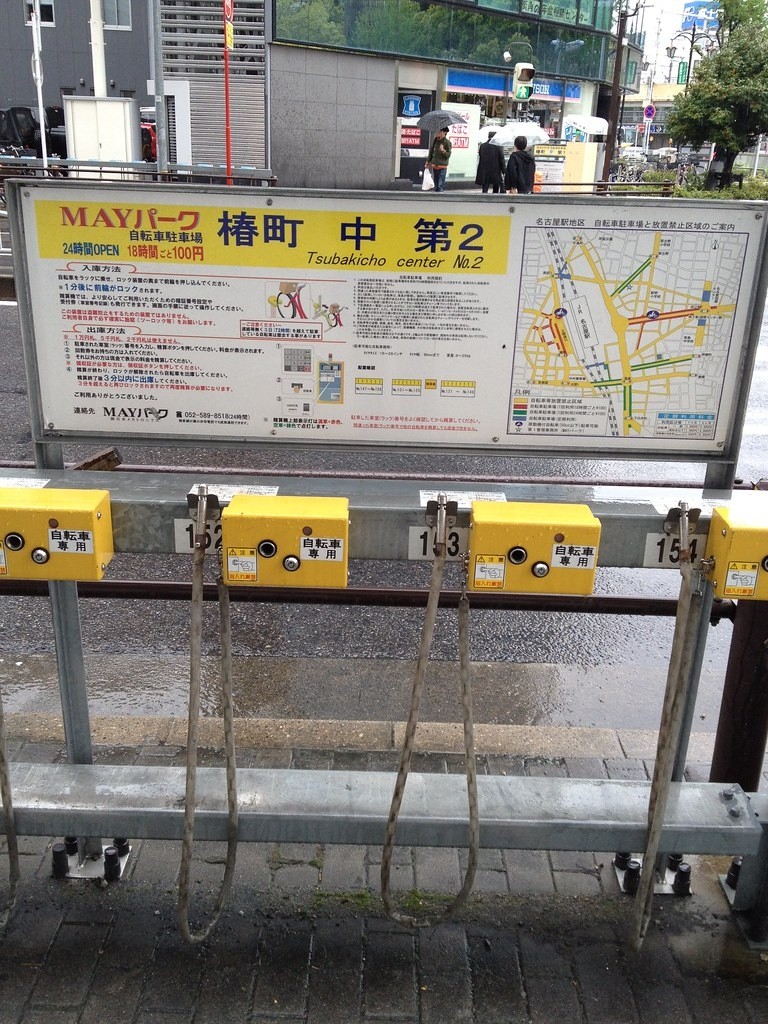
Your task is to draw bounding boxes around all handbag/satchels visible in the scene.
[422,168,435,190]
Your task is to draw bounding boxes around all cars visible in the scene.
[608,145,699,182]
[0,106,157,179]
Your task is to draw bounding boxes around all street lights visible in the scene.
[503,41,533,111]
[666,20,719,166]
[551,37,584,74]
[618,54,641,150]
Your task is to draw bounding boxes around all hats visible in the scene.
[440,127,449,132]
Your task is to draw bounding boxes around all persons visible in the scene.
[504,136,535,194]
[424,127,452,191]
[475,132,507,193]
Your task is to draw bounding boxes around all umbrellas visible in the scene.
[489,122,551,147]
[472,126,503,143]
[416,109,468,144]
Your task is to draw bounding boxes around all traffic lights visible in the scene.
[513,62,535,102]
